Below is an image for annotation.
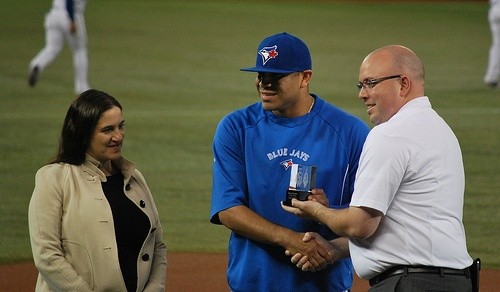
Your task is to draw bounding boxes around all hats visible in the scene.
[239,32,312,74]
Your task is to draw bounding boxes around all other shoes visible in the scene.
[28,65,39,85]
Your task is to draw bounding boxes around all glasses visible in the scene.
[356,74,404,91]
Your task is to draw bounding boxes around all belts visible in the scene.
[368,266,471,287]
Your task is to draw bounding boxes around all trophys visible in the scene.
[285,162,316,207]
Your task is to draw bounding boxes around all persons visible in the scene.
[484,0,500,86]
[280,44,474,292]
[209,32,370,292]
[28,0,90,96]
[28,88,168,292]
